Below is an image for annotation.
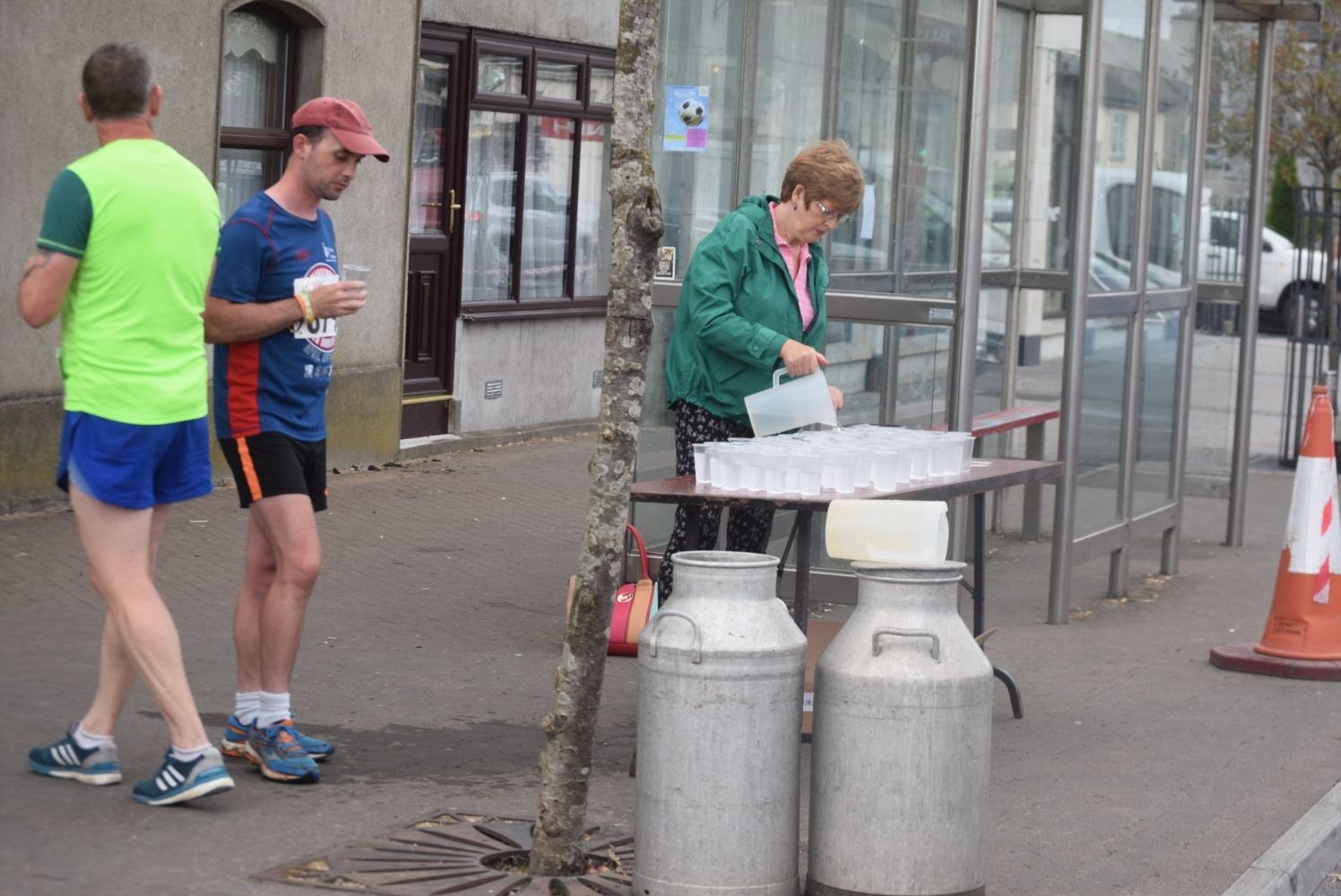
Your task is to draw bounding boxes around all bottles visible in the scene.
[631,549,809,896]
[805,560,995,896]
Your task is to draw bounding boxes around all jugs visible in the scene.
[743,361,837,436]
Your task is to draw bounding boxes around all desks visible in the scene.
[626,459,1067,779]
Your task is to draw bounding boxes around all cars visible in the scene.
[818,161,1186,345]
[464,169,596,288]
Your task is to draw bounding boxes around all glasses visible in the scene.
[814,200,853,224]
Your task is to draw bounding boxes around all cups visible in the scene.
[691,424,977,497]
[342,264,371,294]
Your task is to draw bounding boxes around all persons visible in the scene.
[203,96,392,784]
[658,136,865,604]
[16,42,236,805]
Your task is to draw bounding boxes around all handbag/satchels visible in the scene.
[568,525,659,656]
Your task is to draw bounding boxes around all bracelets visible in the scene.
[295,293,315,323]
[22,263,44,281]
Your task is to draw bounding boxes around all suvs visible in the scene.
[1194,211,1341,342]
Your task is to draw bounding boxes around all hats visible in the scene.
[290,96,390,163]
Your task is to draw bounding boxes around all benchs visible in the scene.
[931,407,1059,540]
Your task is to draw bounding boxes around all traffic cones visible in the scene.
[1209,384,1341,683]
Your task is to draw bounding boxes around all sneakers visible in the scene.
[220,714,334,759]
[243,717,320,783]
[27,722,123,786]
[131,745,236,806]
